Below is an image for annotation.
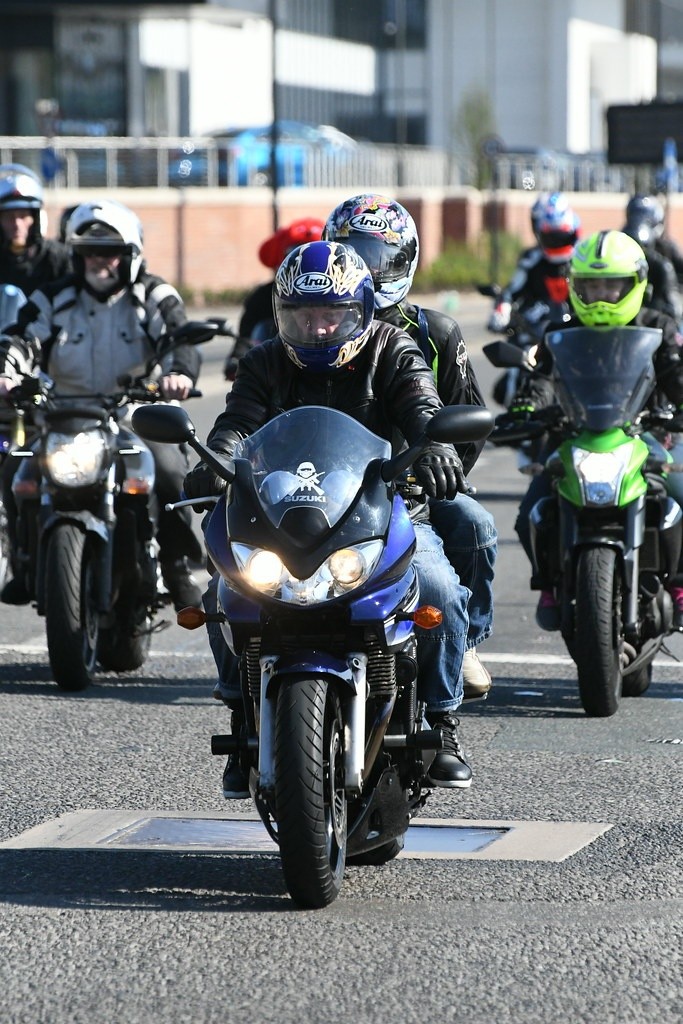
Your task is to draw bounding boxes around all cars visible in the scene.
[161,116,383,189]
[40,116,127,189]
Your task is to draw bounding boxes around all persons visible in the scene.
[182,189,499,786]
[0,164,201,617]
[486,186,683,621]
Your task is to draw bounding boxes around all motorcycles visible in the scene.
[0,265,683,689]
[129,395,497,912]
[481,322,683,720]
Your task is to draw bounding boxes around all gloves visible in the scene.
[184,448,232,512]
[411,439,465,503]
[490,304,512,332]
[505,395,538,428]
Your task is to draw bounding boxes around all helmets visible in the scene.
[532,193,583,264]
[324,189,419,310]
[66,197,142,291]
[625,196,665,237]
[0,163,47,243]
[568,229,649,331]
[622,220,657,250]
[273,241,374,374]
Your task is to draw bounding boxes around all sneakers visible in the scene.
[223,711,252,798]
[0,573,34,605]
[669,586,683,630]
[464,644,491,692]
[427,711,472,790]
[535,589,560,632]
[159,551,202,613]
